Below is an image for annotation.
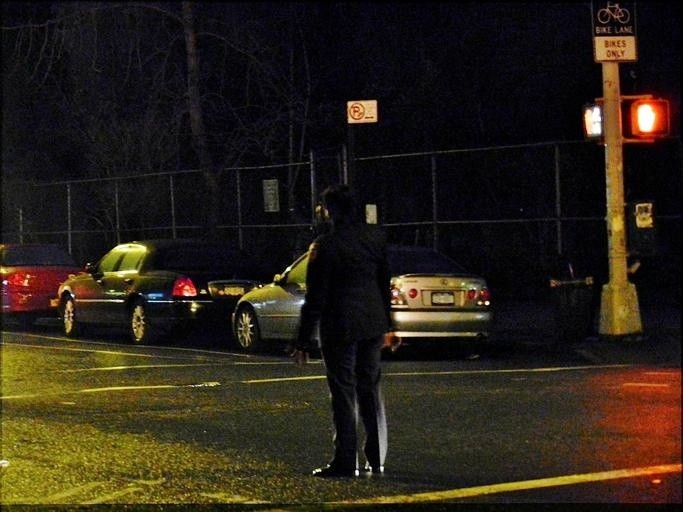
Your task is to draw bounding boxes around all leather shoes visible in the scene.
[310,464,363,478]
[365,460,385,474]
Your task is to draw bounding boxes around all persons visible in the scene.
[292,182,403,479]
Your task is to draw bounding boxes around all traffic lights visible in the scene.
[582,94,670,145]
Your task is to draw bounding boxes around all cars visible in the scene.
[55,239,261,345]
[0,244,83,327]
[231,245,492,356]
